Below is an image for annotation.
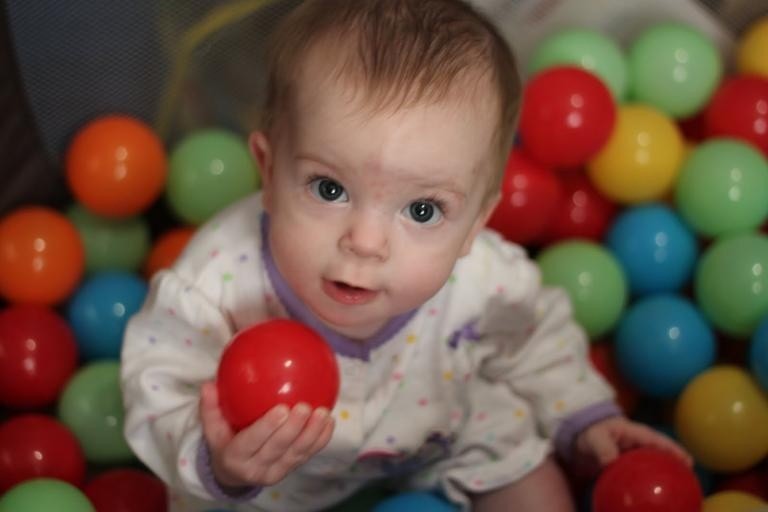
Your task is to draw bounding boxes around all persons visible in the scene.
[120,2,693,511]
